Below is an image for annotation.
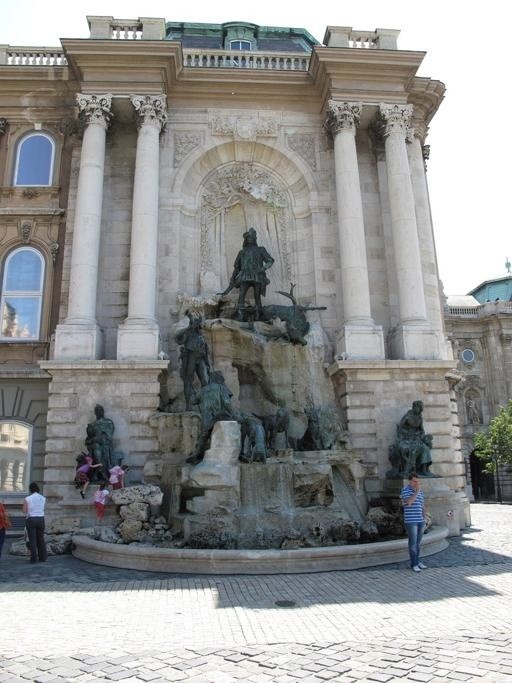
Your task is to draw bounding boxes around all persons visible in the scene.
[192,367,248,459]
[92,480,112,523]
[396,398,435,478]
[465,394,481,424]
[75,455,103,498]
[215,228,275,322]
[18,481,48,562]
[398,471,428,572]
[92,403,115,469]
[107,464,130,489]
[87,423,104,464]
[0,499,11,547]
[173,312,211,411]
[23,523,33,554]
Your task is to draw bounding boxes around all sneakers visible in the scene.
[80,490,86,499]
[413,562,427,572]
